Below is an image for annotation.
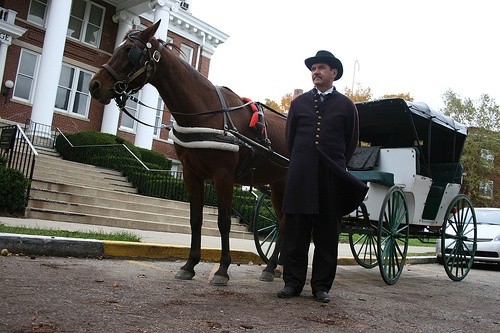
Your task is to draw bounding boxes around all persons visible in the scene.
[277,50,370,303]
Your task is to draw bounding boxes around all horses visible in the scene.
[89,19,290,286]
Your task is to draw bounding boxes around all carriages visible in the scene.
[89,19,477,286]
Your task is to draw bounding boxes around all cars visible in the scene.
[436,206,500,268]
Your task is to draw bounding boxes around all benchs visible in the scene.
[348,170,395,186]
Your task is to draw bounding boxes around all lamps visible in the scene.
[2,80,14,104]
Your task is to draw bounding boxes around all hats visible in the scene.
[305,51,343,81]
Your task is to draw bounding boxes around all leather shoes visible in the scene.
[313,290,330,302]
[277,286,300,298]
